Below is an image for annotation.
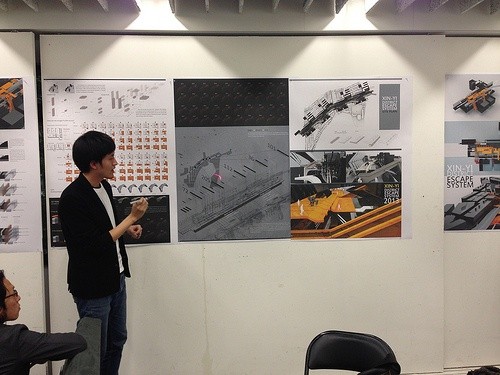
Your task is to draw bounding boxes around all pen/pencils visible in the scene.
[130,197,151,206]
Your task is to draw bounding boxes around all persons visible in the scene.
[56,130,150,375]
[0,269,88,375]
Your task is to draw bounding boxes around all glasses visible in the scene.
[4,290,18,299]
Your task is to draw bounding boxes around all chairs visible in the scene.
[304,330,400,375]
